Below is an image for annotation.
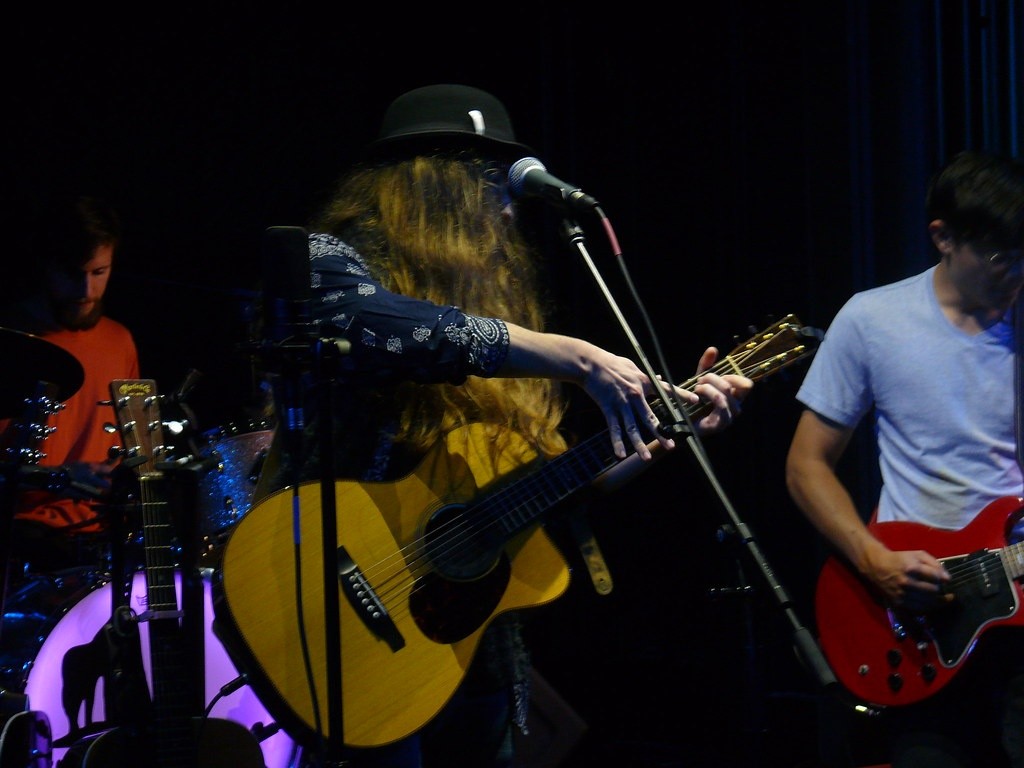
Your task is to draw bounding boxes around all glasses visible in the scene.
[958,229,1023,269]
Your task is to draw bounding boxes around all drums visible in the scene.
[192,415,286,569]
[0,566,306,768]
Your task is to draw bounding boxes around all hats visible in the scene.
[378,83,529,152]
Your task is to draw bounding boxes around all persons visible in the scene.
[249,86,757,768]
[784,158,1023,767]
[0,205,143,581]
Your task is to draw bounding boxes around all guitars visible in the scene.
[816,494,1024,708]
[0,380,65,600]
[57,378,268,768]
[220,315,819,749]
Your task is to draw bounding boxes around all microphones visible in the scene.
[507,156,600,210]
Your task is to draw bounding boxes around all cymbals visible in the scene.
[0,326,86,423]
